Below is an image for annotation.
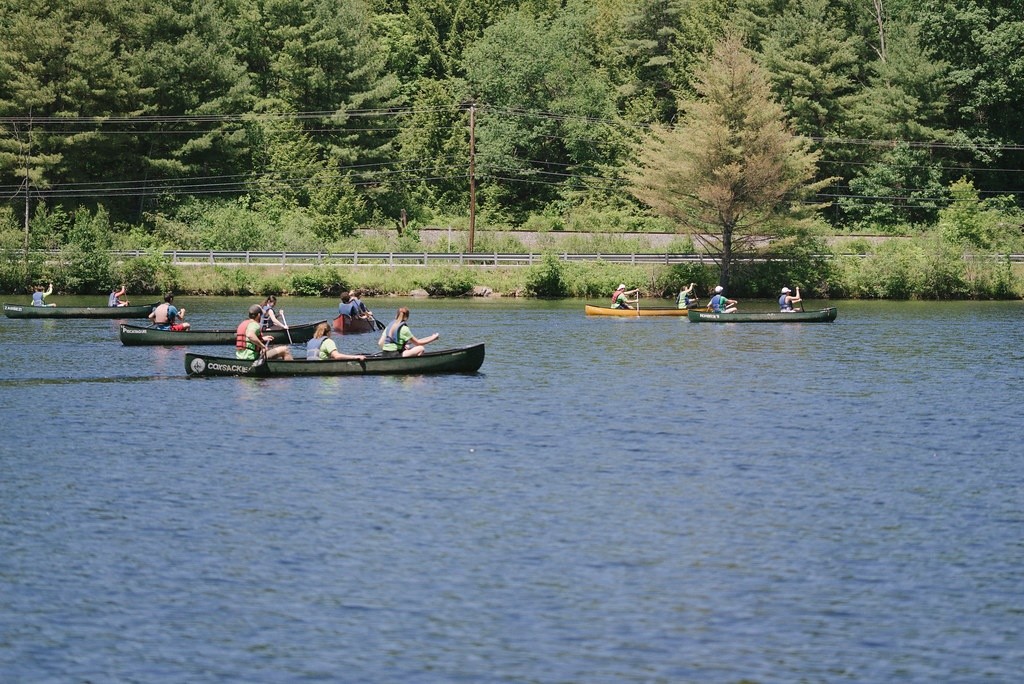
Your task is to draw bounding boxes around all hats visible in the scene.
[617,284,625,291]
[781,287,791,294]
[715,286,723,293]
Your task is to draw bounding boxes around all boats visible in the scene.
[585,304,714,316]
[333,313,374,335]
[3,300,161,319]
[688,307,837,323]
[185,342,485,377]
[120,320,328,346]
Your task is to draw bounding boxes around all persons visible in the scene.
[307,323,366,361]
[33,284,56,307]
[236,304,293,360]
[676,283,698,309]
[611,284,639,309]
[108,286,128,307]
[149,294,190,331]
[339,290,373,320]
[260,296,289,330]
[707,286,737,313]
[378,307,439,357]
[779,287,802,312]
